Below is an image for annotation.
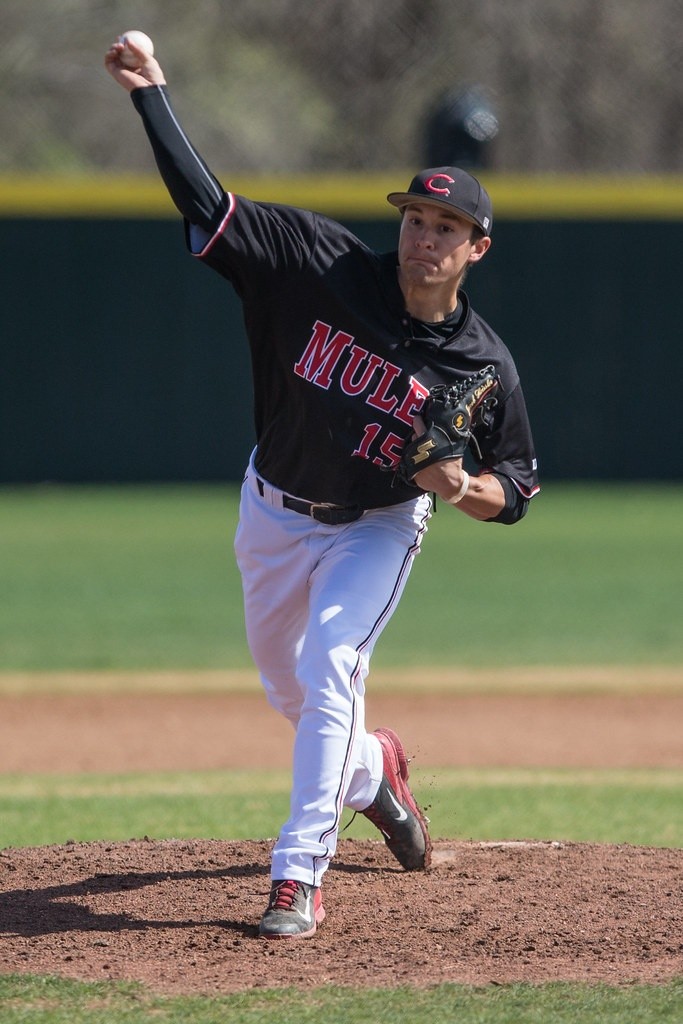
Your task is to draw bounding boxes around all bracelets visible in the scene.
[440,470,469,505]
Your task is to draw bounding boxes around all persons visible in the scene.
[108,33,553,939]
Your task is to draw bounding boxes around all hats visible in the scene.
[387,164,494,234]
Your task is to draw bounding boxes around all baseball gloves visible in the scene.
[400,363,500,480]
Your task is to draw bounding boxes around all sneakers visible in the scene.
[259,879,326,939]
[358,726,431,872]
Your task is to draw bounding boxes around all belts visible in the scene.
[255,478,366,523]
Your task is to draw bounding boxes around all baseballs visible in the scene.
[118,30,154,68]
